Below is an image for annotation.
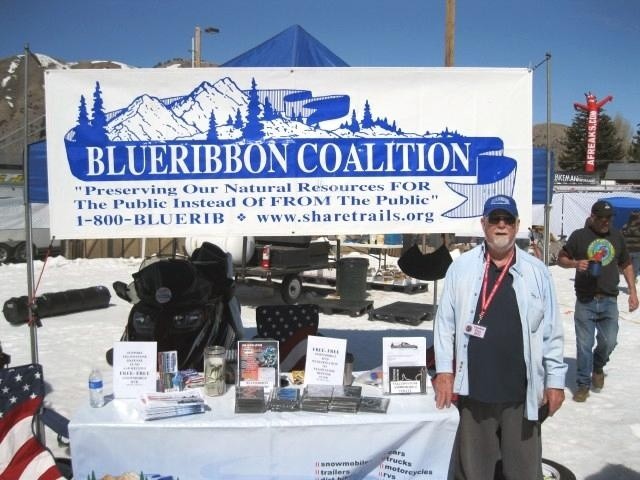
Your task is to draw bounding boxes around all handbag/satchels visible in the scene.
[398,244,454,280]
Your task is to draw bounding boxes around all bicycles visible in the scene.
[524,228,567,266]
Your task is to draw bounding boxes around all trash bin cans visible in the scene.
[598,197,640,228]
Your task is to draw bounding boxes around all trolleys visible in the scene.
[170,237,341,305]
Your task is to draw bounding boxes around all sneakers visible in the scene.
[591,364,604,387]
[574,387,589,401]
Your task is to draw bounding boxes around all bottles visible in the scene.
[172,371,187,391]
[88,366,105,408]
[371,370,383,381]
[343,352,354,386]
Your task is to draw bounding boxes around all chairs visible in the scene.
[0,353,70,480]
[252,304,324,372]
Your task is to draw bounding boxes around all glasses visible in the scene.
[487,215,515,225]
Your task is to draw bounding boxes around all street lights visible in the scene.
[190,24,222,67]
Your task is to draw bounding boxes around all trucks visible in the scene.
[0,228,64,266]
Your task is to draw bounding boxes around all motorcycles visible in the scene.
[112,241,243,388]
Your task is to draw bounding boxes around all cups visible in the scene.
[587,260,602,278]
[203,344,227,398]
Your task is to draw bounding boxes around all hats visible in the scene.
[591,201,615,216]
[483,195,518,218]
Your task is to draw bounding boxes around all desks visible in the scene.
[68,372,460,480]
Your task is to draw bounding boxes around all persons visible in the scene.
[433,191,569,480]
[556,199,640,403]
